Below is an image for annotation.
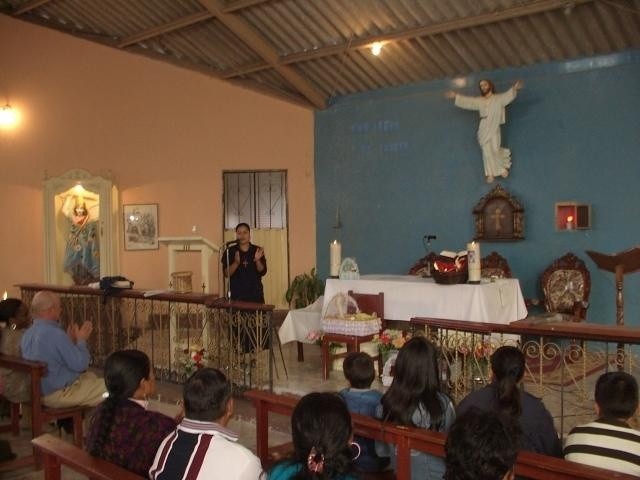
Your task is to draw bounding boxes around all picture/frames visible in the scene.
[123,203,159,250]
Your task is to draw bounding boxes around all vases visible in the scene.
[175,385,185,405]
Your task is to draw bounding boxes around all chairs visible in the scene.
[409,258,448,277]
[321,289,385,381]
[479,253,511,283]
[527,252,592,362]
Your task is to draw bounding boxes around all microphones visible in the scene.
[226,239,240,246]
[424,235,437,240]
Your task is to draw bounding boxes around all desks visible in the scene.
[323,271,528,386]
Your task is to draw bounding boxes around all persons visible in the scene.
[562,370,640,478]
[456,346,562,458]
[266,392,387,480]
[221,223,270,353]
[373,336,456,480]
[332,351,393,469]
[445,78,526,184]
[61,194,100,287]
[20,290,110,447]
[148,368,267,480]
[81,349,178,478]
[438,409,519,480]
[0,298,73,436]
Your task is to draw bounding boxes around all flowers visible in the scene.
[173,344,204,383]
[374,325,495,387]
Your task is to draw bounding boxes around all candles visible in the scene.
[329,238,342,279]
[566,215,574,229]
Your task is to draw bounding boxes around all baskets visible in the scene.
[170,271,193,292]
[322,292,381,337]
[339,257,360,281]
[431,270,469,284]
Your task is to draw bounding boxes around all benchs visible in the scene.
[245,385,638,479]
[0,353,103,471]
[31,433,146,480]
